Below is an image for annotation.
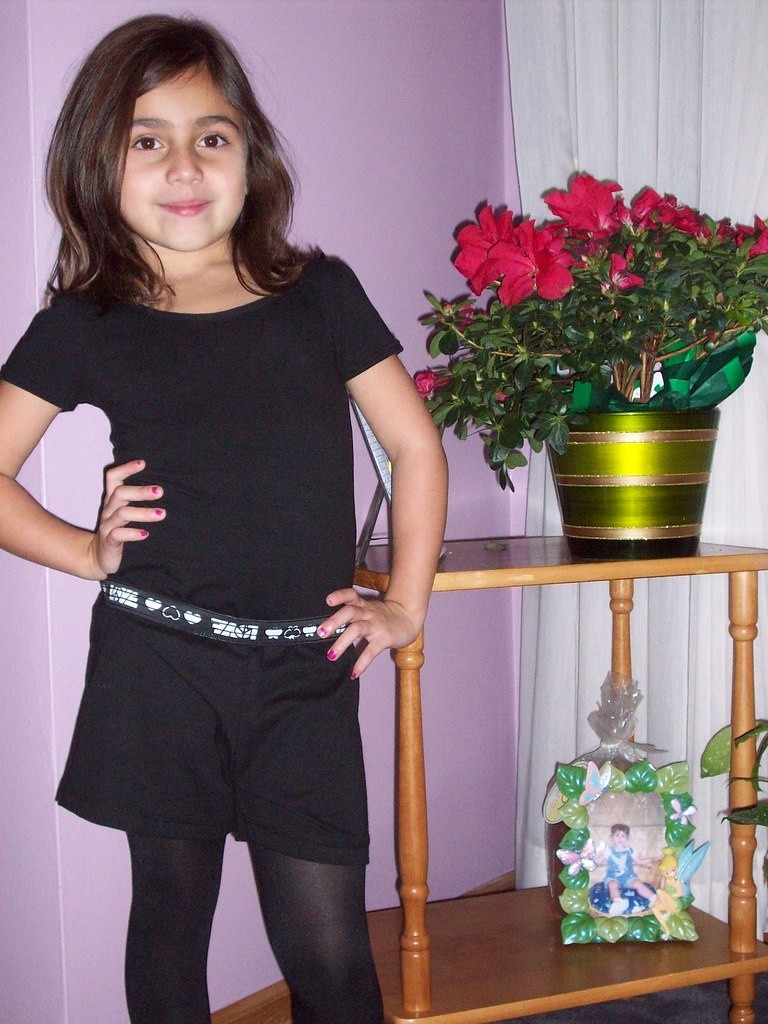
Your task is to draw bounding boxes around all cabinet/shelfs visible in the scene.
[356,538,768,1024]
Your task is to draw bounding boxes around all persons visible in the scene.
[592,824,657,916]
[0,12,449,1024]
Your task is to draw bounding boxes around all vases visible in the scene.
[545,410,720,560]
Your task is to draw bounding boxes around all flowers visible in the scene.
[412,173,768,494]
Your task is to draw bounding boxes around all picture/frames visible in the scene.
[542,760,710,946]
